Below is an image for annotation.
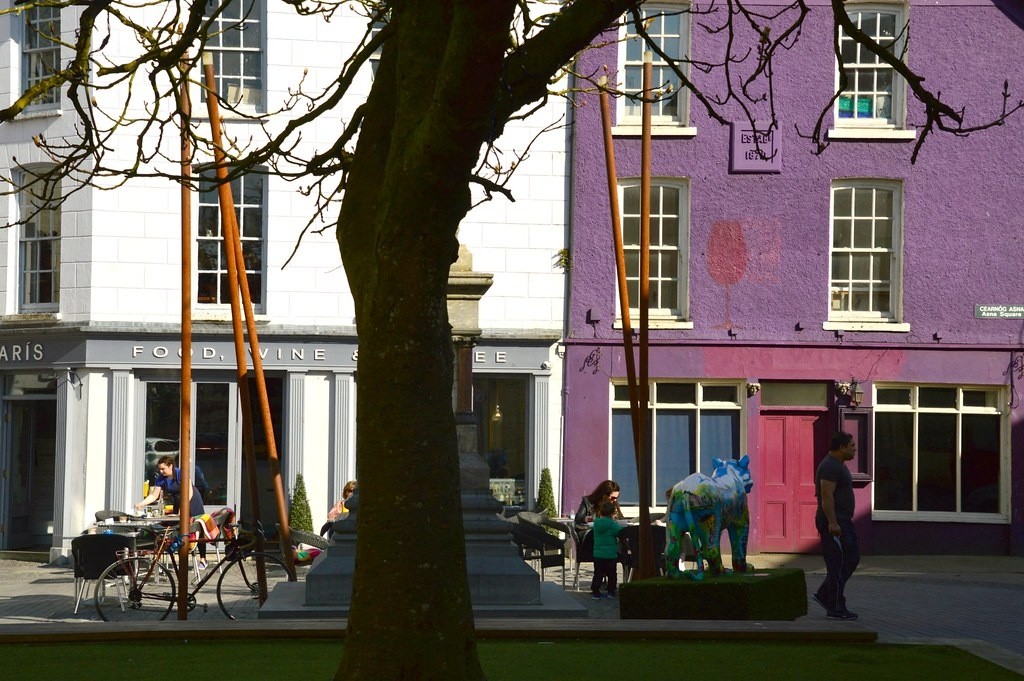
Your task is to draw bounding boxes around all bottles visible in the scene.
[493,483,525,507]
[87,522,113,534]
[157,490,165,517]
[569,509,576,521]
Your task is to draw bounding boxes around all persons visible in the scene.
[811,431,861,621]
[574,480,626,600]
[650,487,706,570]
[320,481,359,543]
[134,455,209,571]
[195,463,209,505]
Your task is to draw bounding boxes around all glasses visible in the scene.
[347,489,353,493]
[608,496,618,501]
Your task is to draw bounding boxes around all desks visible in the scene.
[588,518,636,585]
[97,517,162,588]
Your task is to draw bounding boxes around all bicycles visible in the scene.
[93,516,297,624]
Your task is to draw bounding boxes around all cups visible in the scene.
[147,510,158,518]
[119,516,127,523]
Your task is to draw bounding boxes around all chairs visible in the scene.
[72,506,233,613]
[507,511,666,591]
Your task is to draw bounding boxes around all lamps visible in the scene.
[849,380,865,407]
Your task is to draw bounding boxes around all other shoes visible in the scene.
[606,582,618,591]
[591,593,607,600]
[825,610,858,620]
[198,559,209,570]
[606,592,617,599]
[599,582,608,591]
[811,590,849,618]
